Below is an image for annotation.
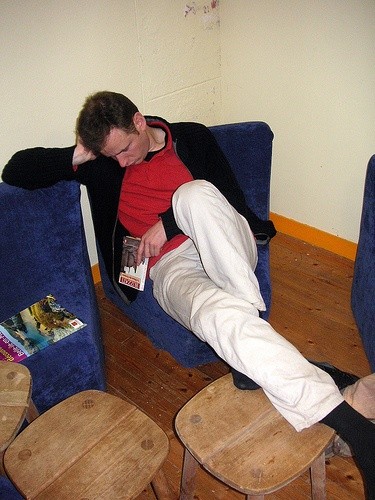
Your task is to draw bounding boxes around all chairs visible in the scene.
[87,121,276,369]
[350,154,375,377]
[0,172,104,410]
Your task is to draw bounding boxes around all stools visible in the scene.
[0,360,46,480]
[174,371,340,500]
[4,389,173,500]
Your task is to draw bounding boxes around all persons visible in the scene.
[1,91,375,500]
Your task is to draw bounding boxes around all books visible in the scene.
[118,236,150,291]
[0,293,88,363]
